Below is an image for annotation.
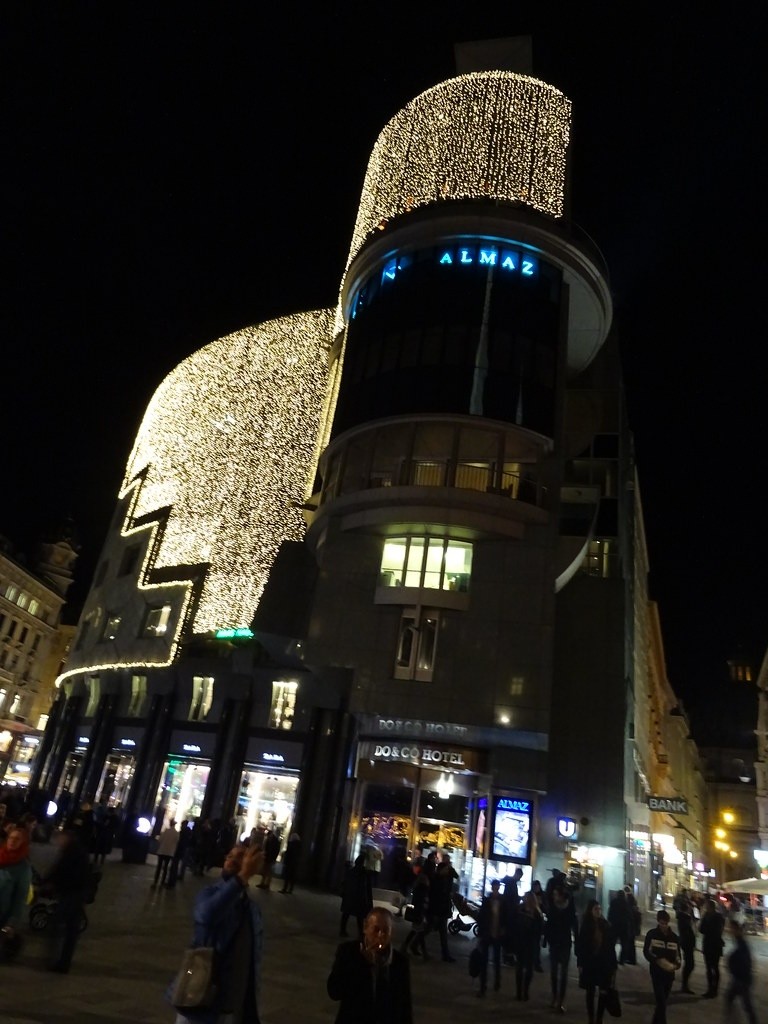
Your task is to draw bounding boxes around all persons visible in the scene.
[607,887,640,965]
[229,818,238,845]
[339,854,373,938]
[662,892,666,906]
[574,900,617,1024]
[643,910,682,1024]
[255,830,280,889]
[150,818,226,889]
[477,868,578,1011]
[0,784,124,974]
[675,903,696,994]
[393,845,459,961]
[326,907,413,1024]
[673,888,747,923]
[698,901,725,998]
[221,832,264,889]
[173,844,266,1024]
[723,920,758,1024]
[277,833,308,894]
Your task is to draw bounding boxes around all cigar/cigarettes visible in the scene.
[378,944,382,949]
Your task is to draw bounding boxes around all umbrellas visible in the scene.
[722,877,768,910]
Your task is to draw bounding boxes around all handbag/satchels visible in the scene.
[606,986,622,1018]
[468,943,485,978]
[169,946,215,1009]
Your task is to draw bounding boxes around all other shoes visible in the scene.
[410,944,421,956]
[337,931,347,937]
[358,933,365,936]
[164,883,175,889]
[442,956,454,961]
[151,884,157,889]
[399,946,409,956]
[158,885,163,890]
[279,889,293,895]
[421,952,435,960]
[256,883,270,888]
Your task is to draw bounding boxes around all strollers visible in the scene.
[449,890,484,938]
[29,865,65,934]
